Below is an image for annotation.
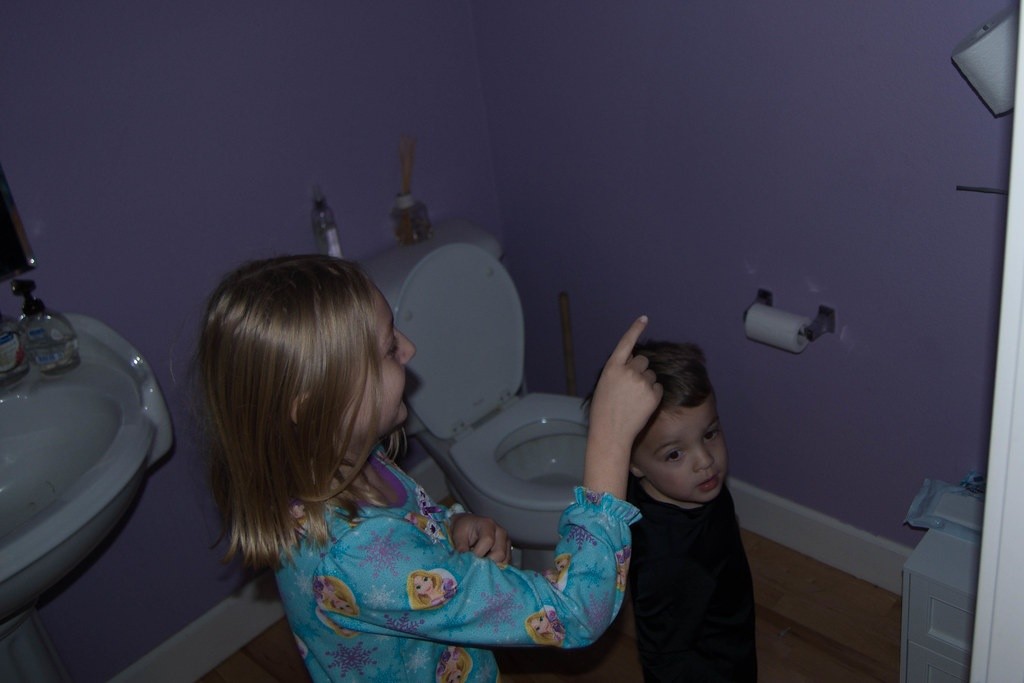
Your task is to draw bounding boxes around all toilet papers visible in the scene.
[744,303,811,353]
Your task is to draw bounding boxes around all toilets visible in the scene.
[363,218,593,574]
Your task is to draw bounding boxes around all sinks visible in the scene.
[0,309,175,622]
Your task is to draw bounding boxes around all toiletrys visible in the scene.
[1,281,83,391]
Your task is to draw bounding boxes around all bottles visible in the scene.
[0,311,30,388]
[389,191,435,246]
[311,182,344,259]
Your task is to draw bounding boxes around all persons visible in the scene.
[191,254,662,683]
[580,341,758,683]
